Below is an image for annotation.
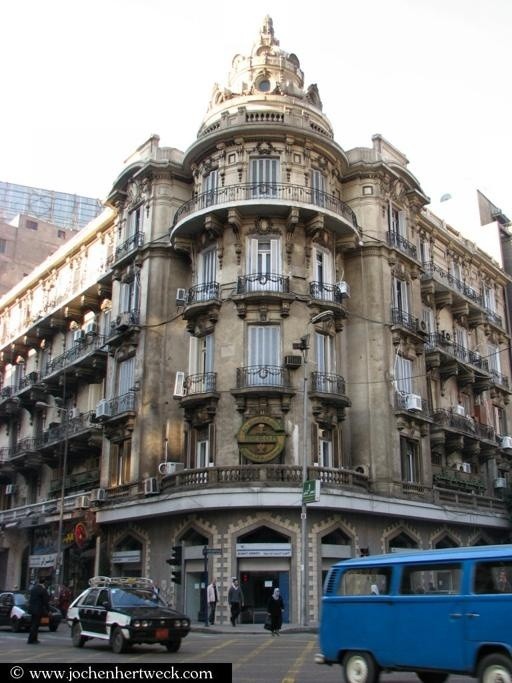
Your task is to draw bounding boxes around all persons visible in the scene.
[268,588,284,636]
[207,578,219,625]
[28,577,49,644]
[228,579,244,627]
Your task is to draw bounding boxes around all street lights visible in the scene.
[34,398,70,602]
[299,304,335,628]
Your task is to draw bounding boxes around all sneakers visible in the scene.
[27,639,41,644]
[271,632,280,637]
[230,617,235,626]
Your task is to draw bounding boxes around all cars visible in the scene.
[63,575,191,654]
[0,589,64,633]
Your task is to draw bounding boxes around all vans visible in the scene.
[312,542,512,682]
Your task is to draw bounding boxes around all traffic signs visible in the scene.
[202,548,222,554]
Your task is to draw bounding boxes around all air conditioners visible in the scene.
[0,288,186,510]
[335,281,512,489]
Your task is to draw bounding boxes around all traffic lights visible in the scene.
[165,545,182,566]
[170,570,182,585]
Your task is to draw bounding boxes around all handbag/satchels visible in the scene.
[264,619,272,630]
